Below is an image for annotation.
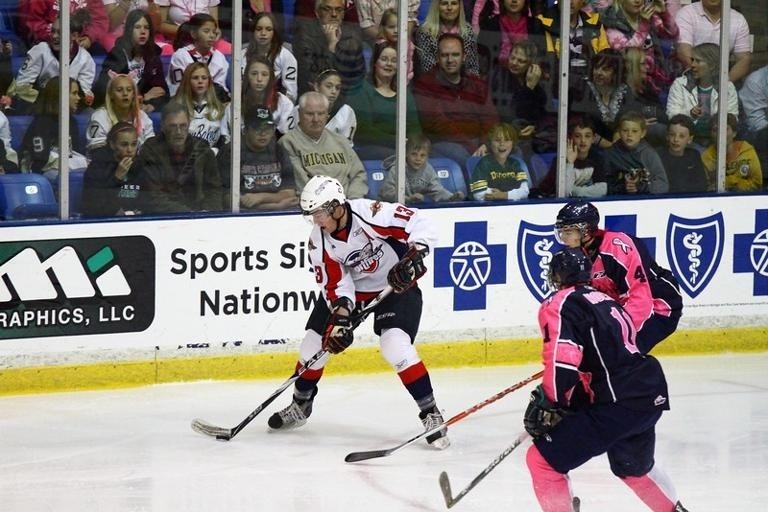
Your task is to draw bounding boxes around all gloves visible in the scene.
[321,313,354,354]
[522,382,566,441]
[386,243,428,296]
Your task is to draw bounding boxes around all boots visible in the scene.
[269,385,320,431]
[418,405,448,445]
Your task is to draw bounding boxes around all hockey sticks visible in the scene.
[440,430,529,508]
[191,284,395,438]
[345,371,545,463]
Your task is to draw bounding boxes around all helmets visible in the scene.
[298,173,347,212]
[553,199,601,238]
[541,248,593,285]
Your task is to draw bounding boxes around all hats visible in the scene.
[243,103,276,127]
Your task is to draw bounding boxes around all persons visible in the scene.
[551,196,683,359]
[1,0,768,222]
[518,248,689,512]
[264,173,452,454]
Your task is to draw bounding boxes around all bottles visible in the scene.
[20,151,32,173]
